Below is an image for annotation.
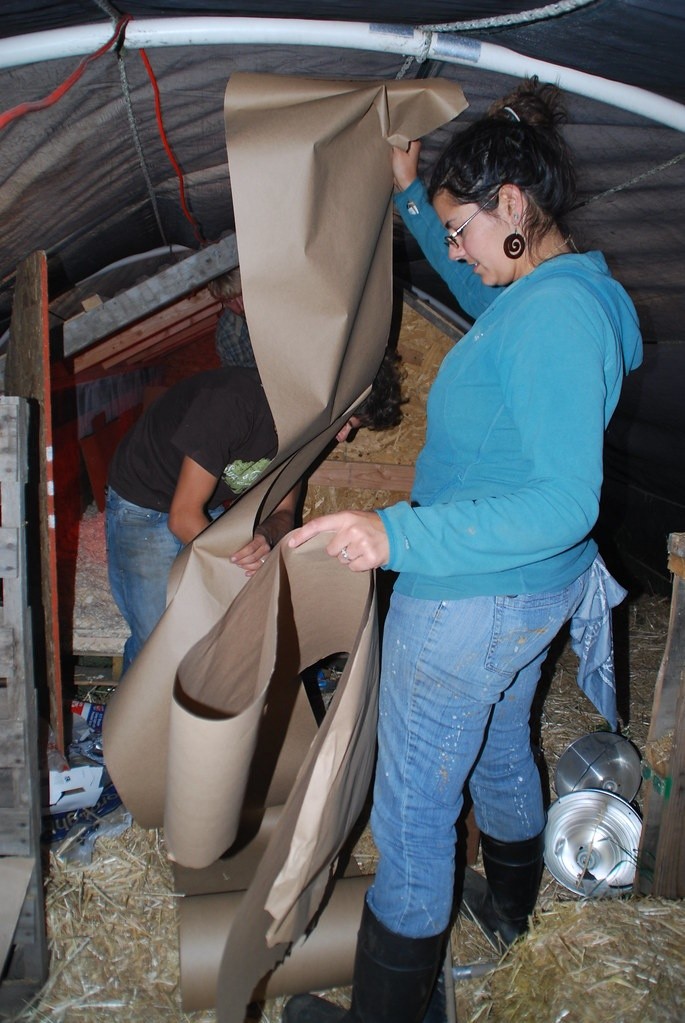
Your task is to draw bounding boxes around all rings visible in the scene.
[260,558,265,563]
[340,548,353,562]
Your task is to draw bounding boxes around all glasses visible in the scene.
[443,192,499,249]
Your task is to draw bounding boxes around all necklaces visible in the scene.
[540,234,580,263]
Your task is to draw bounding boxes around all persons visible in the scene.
[103,346,407,691]
[287,74,643,1023]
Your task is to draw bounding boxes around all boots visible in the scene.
[453,811,549,957]
[281,891,451,1023]
